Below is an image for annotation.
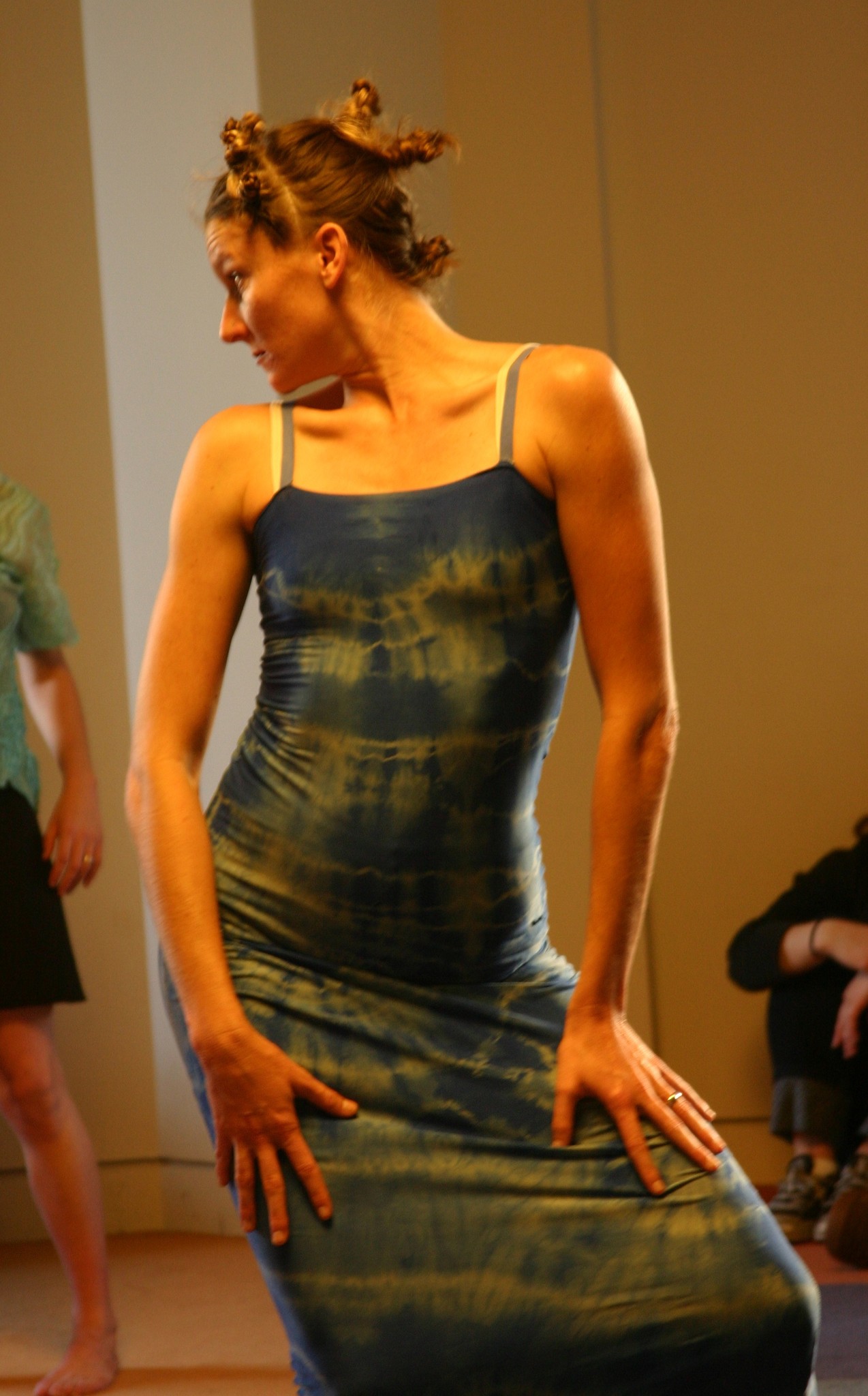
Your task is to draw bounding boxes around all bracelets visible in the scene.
[808,918,832,958]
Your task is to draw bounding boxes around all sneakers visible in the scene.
[766,1152,868,1249]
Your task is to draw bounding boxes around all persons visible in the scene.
[723,811,868,1268]
[0,467,123,1396]
[119,75,828,1396]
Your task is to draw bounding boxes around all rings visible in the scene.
[82,854,96,864]
[664,1091,683,1107]
